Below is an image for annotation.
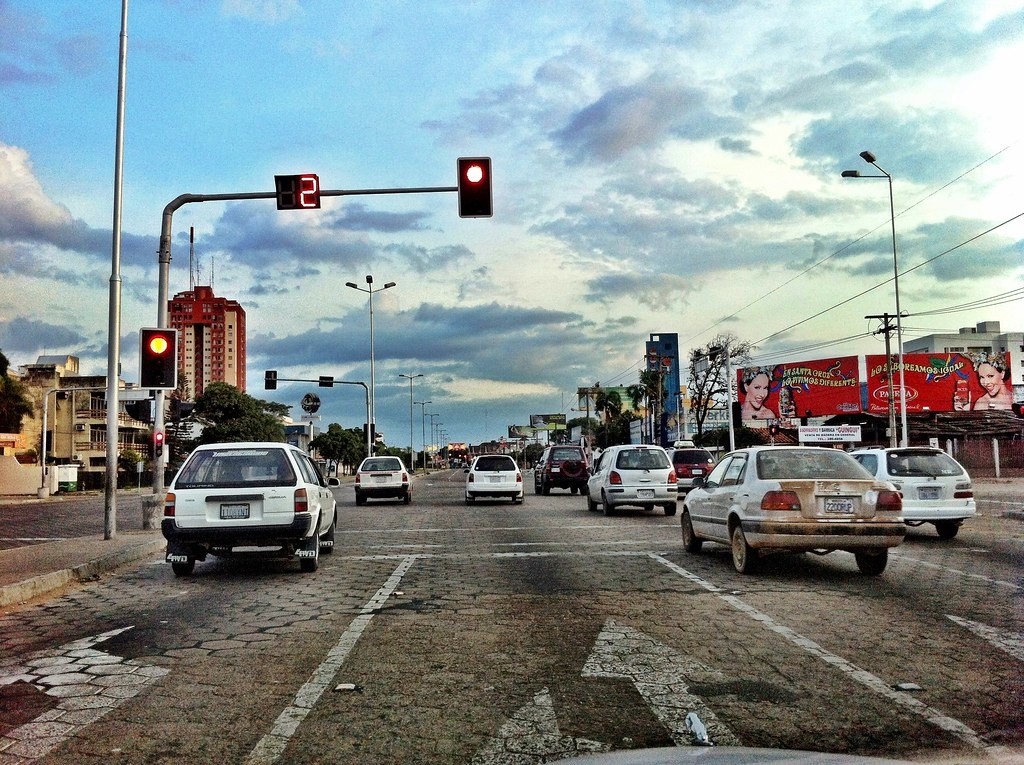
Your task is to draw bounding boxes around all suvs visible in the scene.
[534,446,591,496]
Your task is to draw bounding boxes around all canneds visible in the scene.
[957,380,969,404]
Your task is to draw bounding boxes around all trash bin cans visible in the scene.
[58,464,79,491]
[44,465,58,494]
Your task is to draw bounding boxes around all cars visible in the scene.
[463,455,524,506]
[665,441,717,496]
[586,444,678,516]
[847,445,976,539]
[162,442,340,578]
[354,456,414,506]
[681,446,910,576]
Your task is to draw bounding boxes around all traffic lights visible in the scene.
[139,327,178,390]
[155,432,164,457]
[1011,401,1024,419]
[124,399,155,424]
[769,424,780,436]
[273,173,321,211]
[456,157,493,218]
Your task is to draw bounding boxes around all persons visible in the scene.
[740,367,776,420]
[591,444,603,468]
[953,351,1014,411]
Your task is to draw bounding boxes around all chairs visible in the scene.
[370,464,378,470]
[217,461,242,482]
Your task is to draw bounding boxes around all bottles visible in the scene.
[780,365,793,415]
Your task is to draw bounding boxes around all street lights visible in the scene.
[425,413,452,471]
[399,373,423,474]
[345,274,396,457]
[413,399,432,470]
[840,150,907,447]
